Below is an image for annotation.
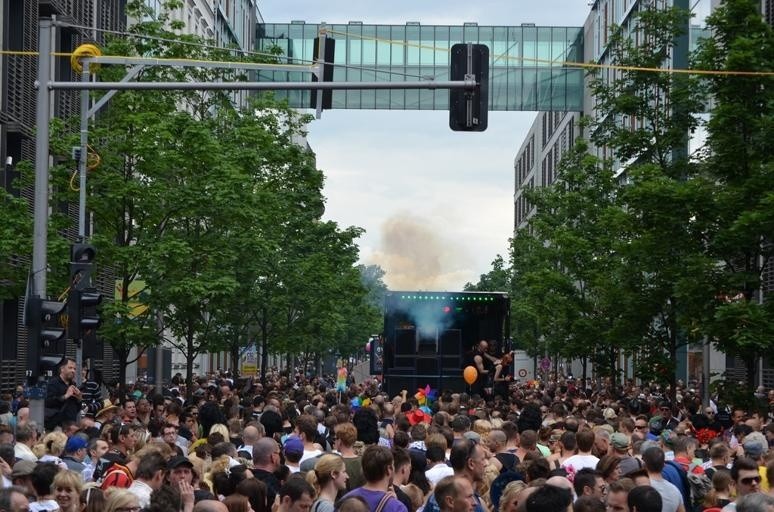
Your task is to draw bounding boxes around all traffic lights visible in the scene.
[68,243,102,341]
[25,295,67,386]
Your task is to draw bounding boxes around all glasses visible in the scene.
[741,476,762,486]
[332,437,339,441]
[660,408,670,412]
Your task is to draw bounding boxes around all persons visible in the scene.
[0,356,774,512]
[465,340,498,393]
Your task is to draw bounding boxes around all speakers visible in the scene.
[440,367,467,392]
[387,367,418,398]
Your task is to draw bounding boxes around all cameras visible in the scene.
[550,435,562,441]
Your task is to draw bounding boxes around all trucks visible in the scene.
[369,288,512,399]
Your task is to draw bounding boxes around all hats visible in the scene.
[169,457,194,473]
[611,432,646,476]
[714,410,731,424]
[65,436,91,451]
[281,434,303,457]
[11,455,60,477]
[658,401,671,408]
[95,399,117,415]
